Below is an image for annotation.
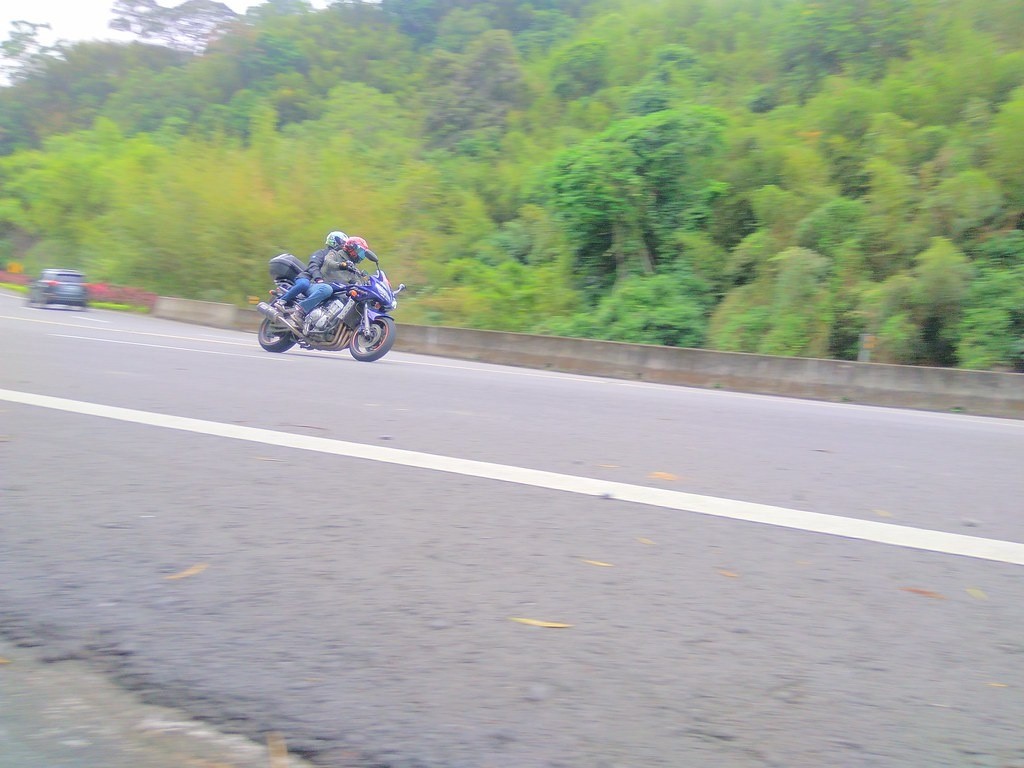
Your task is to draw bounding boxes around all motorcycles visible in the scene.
[255,249,405,362]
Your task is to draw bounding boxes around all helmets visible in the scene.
[344,236,368,264]
[325,232,349,251]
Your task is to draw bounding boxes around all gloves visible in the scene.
[343,260,354,267]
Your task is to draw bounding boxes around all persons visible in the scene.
[275,231,368,330]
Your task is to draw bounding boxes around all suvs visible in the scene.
[26,268,88,309]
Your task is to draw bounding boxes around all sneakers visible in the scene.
[291,312,305,328]
[273,299,287,314]
